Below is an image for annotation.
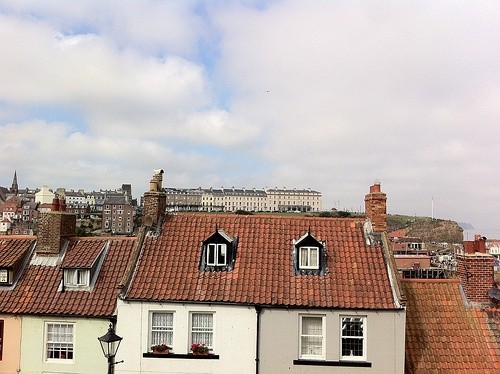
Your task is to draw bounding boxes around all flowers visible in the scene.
[150,343,172,353]
[190,343,209,353]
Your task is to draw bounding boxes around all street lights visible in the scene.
[97,322,125,374]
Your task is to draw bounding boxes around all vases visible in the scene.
[192,350,209,356]
[152,349,169,354]
